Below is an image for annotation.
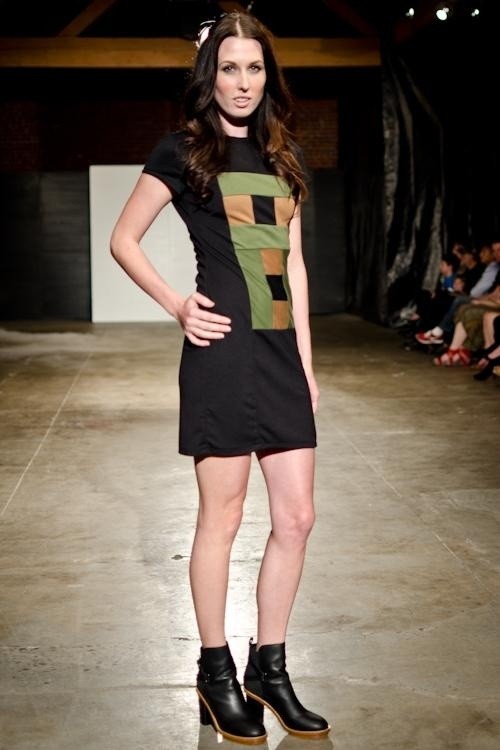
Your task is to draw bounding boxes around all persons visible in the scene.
[398,233,500,382]
[108,8,334,743]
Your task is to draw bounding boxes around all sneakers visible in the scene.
[492,366,500,376]
[402,329,471,367]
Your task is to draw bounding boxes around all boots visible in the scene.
[244,638,331,739]
[196,640,267,744]
